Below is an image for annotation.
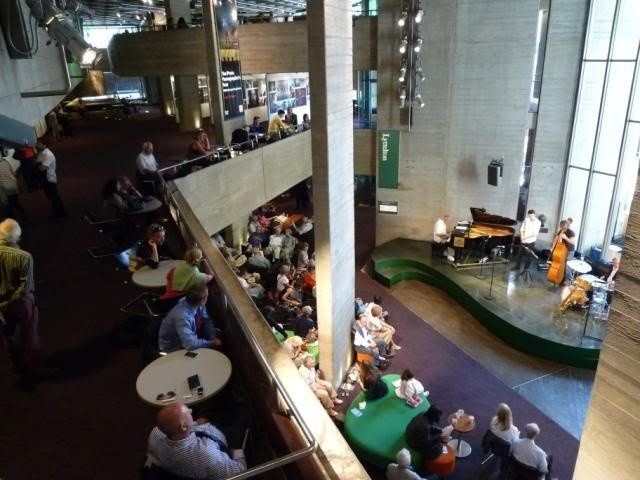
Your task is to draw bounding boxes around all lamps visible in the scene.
[394,0,425,111]
[24,0,98,70]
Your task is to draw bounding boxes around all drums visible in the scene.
[561,274,614,312]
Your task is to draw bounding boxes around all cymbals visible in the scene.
[566,260,592,273]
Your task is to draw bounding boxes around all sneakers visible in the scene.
[331,397,344,405]
[329,409,340,417]
[391,345,402,350]
[423,390,430,397]
[443,251,455,262]
[379,354,395,364]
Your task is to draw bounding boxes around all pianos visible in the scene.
[445,208,517,260]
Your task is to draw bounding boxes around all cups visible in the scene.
[468,415,475,424]
[452,409,464,426]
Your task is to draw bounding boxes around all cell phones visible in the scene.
[184,351,200,359]
[197,385,204,397]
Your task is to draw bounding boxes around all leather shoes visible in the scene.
[511,266,520,271]
[520,271,528,275]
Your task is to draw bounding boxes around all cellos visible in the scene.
[547,229,569,284]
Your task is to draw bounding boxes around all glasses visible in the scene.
[155,390,177,401]
[151,225,164,235]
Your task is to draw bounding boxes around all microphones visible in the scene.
[497,245,506,249]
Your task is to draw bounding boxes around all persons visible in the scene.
[211,201,343,416]
[268,107,298,142]
[230,116,268,154]
[158,246,215,300]
[36,141,62,200]
[187,131,212,168]
[136,140,163,184]
[550,219,576,287]
[302,114,309,131]
[136,223,180,268]
[406,403,452,456]
[351,296,402,400]
[433,212,463,268]
[399,367,418,406]
[567,218,573,226]
[144,402,248,480]
[386,447,438,480]
[47,99,90,144]
[585,257,619,309]
[510,209,541,272]
[0,218,44,391]
[157,282,223,353]
[508,422,549,480]
[101,174,143,247]
[490,403,519,444]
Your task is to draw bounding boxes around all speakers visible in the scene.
[488,164,500,187]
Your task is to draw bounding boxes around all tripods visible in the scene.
[515,252,533,282]
